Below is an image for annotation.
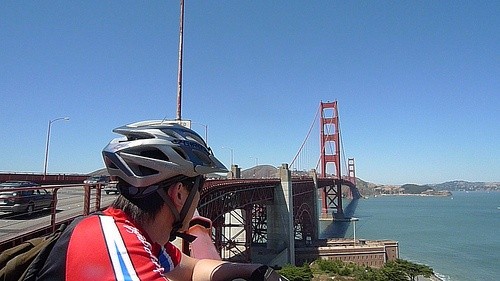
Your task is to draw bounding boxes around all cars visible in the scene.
[104,180,119,194]
[0,180,56,219]
[84,175,107,188]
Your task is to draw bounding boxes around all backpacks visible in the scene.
[0,212,105,281]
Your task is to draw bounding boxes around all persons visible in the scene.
[35,119,283,281]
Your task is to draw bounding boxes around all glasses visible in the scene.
[182,178,206,192]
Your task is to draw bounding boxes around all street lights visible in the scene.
[221,146,233,180]
[250,155,258,166]
[44,117,69,174]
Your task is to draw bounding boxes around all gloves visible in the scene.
[250,265,290,281]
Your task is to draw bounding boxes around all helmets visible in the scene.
[102,119,230,199]
